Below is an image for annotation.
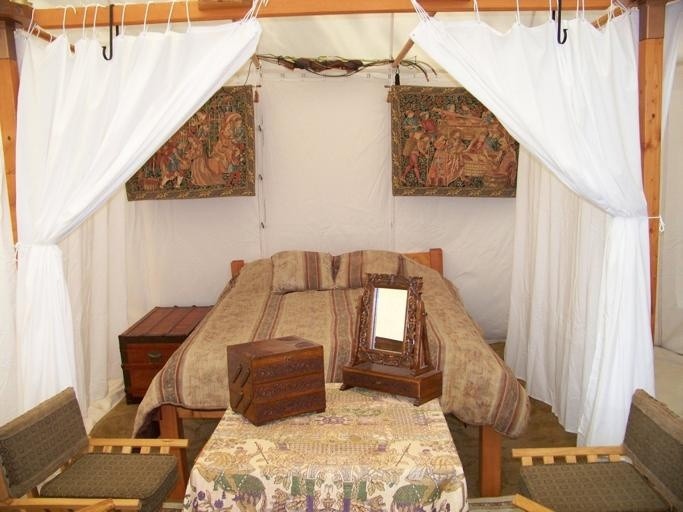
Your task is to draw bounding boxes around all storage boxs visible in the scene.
[226,335,326,426]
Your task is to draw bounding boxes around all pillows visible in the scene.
[267,250,410,294]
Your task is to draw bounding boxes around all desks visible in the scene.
[183,381,469,512]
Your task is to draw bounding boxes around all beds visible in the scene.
[132,250,531,498]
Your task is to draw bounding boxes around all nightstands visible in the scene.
[117,305,212,405]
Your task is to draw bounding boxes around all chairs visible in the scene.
[0,387,188,512]
[509,389,683,512]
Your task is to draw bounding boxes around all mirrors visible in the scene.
[353,273,429,374]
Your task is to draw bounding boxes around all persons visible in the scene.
[400,101,516,191]
[131,108,248,188]
